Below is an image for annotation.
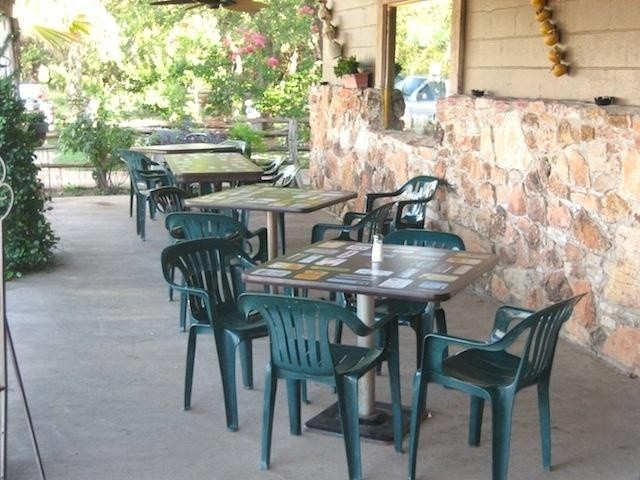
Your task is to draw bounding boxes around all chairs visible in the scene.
[311,202,396,243]
[166,212,267,331]
[218,140,252,159]
[238,292,402,479]
[118,149,187,242]
[161,237,298,431]
[366,176,439,243]
[241,164,302,255]
[408,292,589,479]
[334,230,465,389]
[263,156,288,176]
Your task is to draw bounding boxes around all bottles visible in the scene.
[370,233,383,263]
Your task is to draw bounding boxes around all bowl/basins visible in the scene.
[594,96,616,105]
[471,89,486,95]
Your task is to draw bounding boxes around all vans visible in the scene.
[395,75,450,134]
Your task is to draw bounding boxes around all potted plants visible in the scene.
[333,54,375,89]
[28,111,49,147]
